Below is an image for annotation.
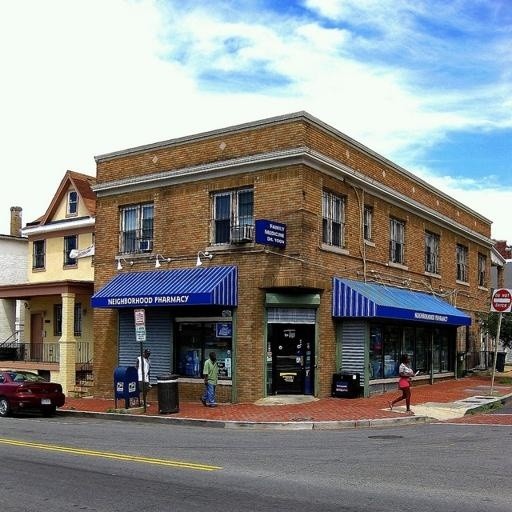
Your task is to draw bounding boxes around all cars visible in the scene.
[0,371,65,419]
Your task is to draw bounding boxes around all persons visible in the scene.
[389,354,416,415]
[135,349,152,409]
[200,353,219,407]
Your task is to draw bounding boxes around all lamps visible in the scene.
[194,250,212,266]
[115,254,134,271]
[154,253,170,269]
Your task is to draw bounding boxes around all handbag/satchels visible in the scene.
[399,377,410,390]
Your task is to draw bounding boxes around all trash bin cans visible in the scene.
[157,375,179,414]
[491,352,507,372]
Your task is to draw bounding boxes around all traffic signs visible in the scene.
[134,309,146,342]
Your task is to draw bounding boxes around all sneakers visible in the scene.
[405,410,414,415]
[388,400,393,410]
[200,397,218,408]
[132,399,151,408]
